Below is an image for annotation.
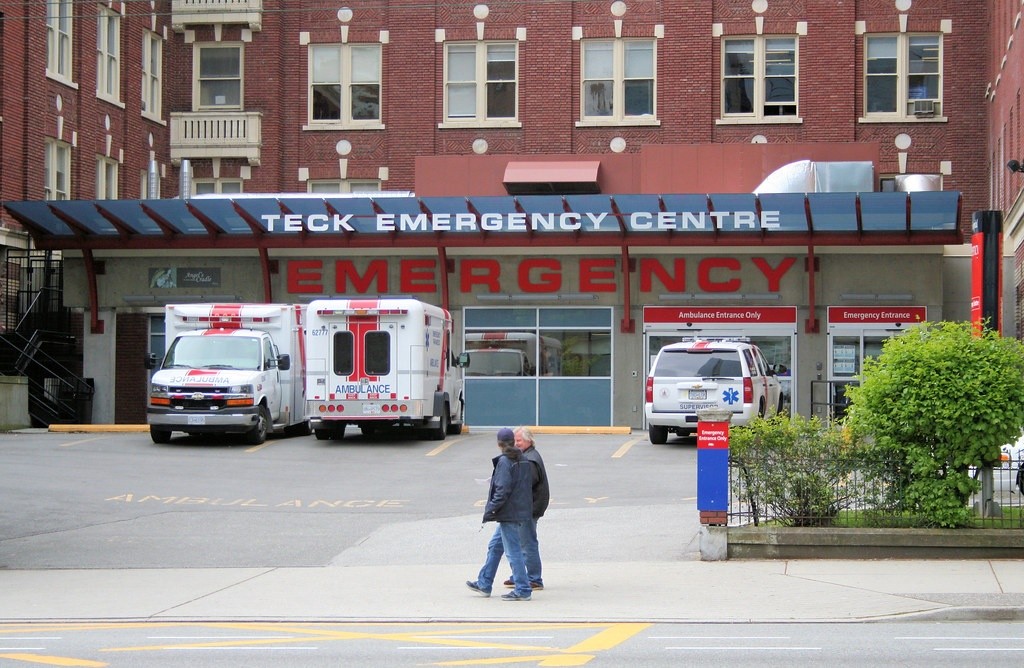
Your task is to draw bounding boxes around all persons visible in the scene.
[465,428,532,601]
[496,427,550,590]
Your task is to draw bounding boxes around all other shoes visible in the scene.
[504,580,516,588]
[530,582,543,590]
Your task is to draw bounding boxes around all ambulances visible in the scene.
[302,296,473,441]
[463,332,563,378]
[642,335,789,446]
[144,299,317,445]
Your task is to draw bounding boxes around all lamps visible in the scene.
[1008,159,1024,173]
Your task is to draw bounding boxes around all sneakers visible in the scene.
[501,591,531,601]
[466,581,490,597]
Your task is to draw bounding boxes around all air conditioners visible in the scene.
[914,100,934,113]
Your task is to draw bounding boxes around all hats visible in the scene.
[497,428,514,440]
